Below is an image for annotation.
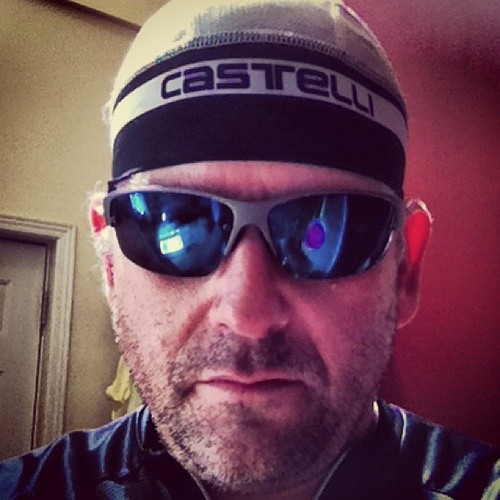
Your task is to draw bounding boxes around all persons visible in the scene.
[0,0,498,498]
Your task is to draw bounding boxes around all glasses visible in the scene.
[101,187,407,280]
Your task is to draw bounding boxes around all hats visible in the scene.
[102,1,409,200]
